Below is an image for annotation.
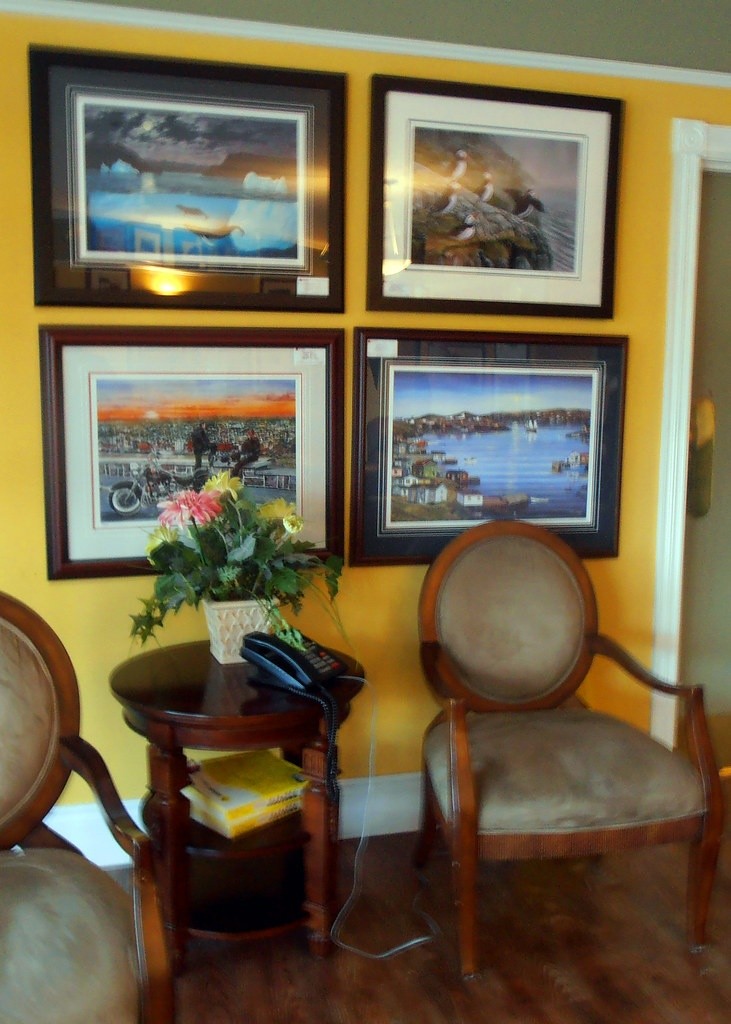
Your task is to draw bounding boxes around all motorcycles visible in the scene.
[109,450,216,519]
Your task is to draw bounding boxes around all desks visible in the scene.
[109,638,366,975]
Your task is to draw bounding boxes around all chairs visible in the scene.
[416,522,724,981]
[0,590,177,1024]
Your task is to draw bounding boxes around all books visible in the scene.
[180,750,310,819]
[189,799,301,838]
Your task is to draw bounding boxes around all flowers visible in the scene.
[128,470,359,674]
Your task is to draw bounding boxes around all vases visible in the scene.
[202,597,280,664]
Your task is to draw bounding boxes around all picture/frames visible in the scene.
[29,41,348,314]
[366,73,624,319]
[350,327,630,567]
[38,325,346,581]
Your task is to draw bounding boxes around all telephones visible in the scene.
[238,629,348,688]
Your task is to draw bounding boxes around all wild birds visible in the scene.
[425,182,459,219]
[476,172,494,203]
[447,149,468,180]
[504,187,545,219]
[445,211,480,241]
